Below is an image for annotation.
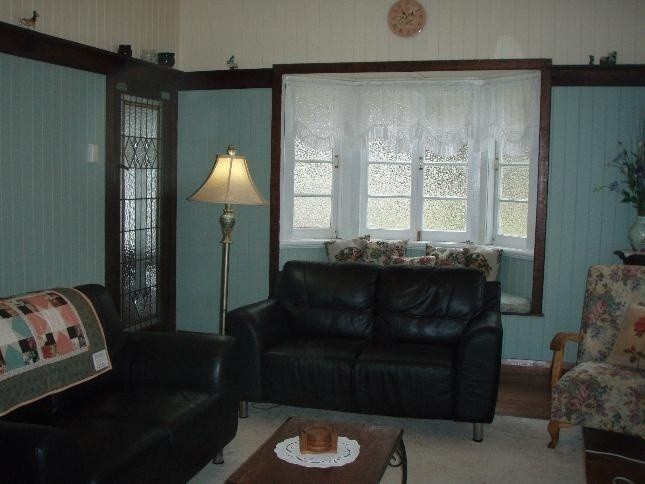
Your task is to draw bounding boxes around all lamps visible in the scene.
[180,144,266,333]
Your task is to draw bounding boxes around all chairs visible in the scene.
[543,261,645,447]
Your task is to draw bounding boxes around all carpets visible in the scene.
[191,396,586,483]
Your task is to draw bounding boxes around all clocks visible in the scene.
[387,0,426,38]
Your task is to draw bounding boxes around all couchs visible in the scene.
[0,282,244,480]
[227,259,498,445]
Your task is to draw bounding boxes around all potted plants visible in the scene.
[593,135,644,255]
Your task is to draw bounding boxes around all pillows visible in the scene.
[606,300,645,371]
[325,234,503,285]
